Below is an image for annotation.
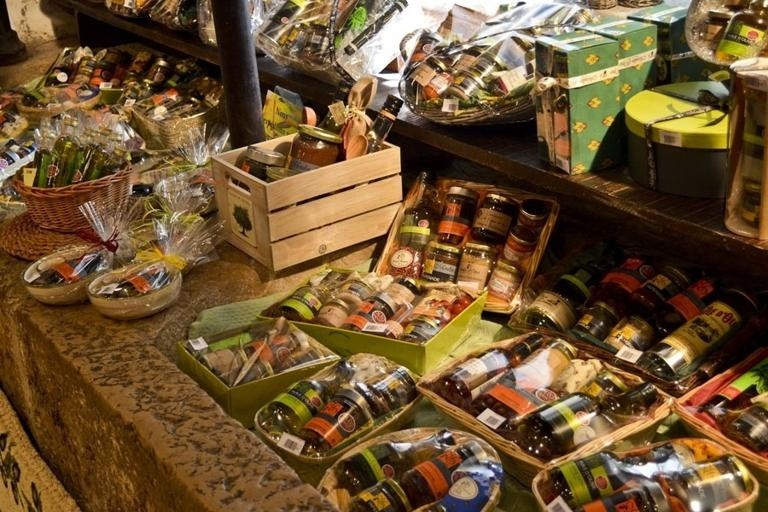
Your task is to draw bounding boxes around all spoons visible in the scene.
[346,135,367,163]
[301,106,318,128]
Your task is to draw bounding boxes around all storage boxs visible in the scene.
[211,131,403,272]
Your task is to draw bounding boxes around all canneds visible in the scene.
[344,440,487,512]
[571,452,752,512]
[403,31,535,107]
[238,124,344,229]
[728,400,767,450]
[316,275,443,346]
[524,250,756,379]
[73,47,225,125]
[510,338,578,389]
[584,371,626,401]
[215,345,324,387]
[420,185,551,303]
[274,20,331,66]
[299,363,417,455]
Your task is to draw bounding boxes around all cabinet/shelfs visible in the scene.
[0,0,768,512]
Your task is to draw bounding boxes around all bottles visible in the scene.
[334,427,458,496]
[276,271,343,323]
[715,0,768,64]
[516,382,660,459]
[365,90,404,156]
[548,441,676,508]
[397,169,444,247]
[44,49,73,88]
[438,333,544,407]
[697,358,768,433]
[258,360,356,441]
